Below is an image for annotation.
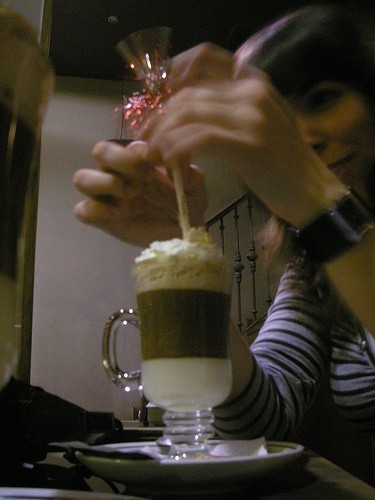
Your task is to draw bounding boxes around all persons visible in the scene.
[72,5,375,490]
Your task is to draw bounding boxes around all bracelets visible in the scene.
[288,185,371,267]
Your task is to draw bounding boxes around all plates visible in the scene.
[75,440,305,484]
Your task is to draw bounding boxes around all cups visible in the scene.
[102,253,232,458]
